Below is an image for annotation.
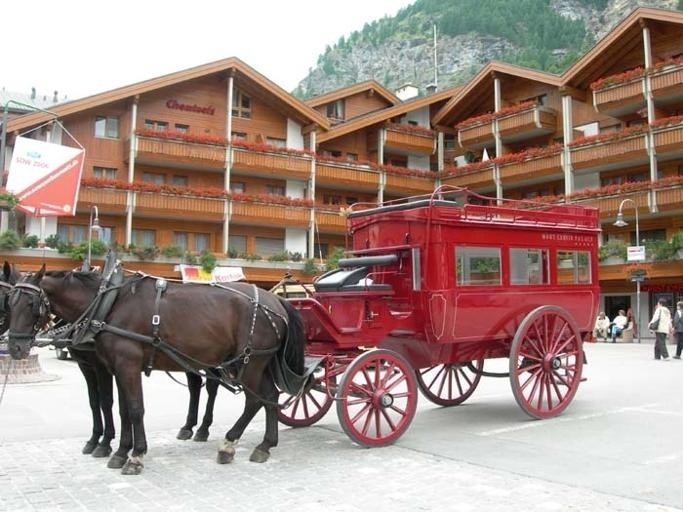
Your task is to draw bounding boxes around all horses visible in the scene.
[7,262,306,475]
[0,260,222,457]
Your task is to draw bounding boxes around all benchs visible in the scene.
[608,321,634,343]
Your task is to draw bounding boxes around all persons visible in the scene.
[671,301,683,359]
[611,310,628,343]
[648,298,672,361]
[593,311,610,343]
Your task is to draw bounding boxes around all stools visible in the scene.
[313,266,368,292]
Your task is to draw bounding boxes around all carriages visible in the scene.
[1,183,604,474]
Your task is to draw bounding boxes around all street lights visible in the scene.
[612,197,641,345]
[87,205,101,269]
